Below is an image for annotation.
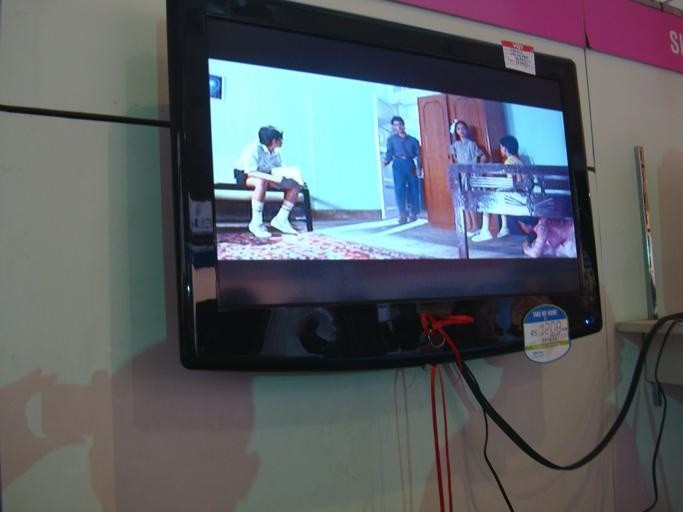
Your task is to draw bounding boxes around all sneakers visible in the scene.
[399,215,416,224]
[270,216,298,235]
[249,222,272,239]
[497,227,510,238]
[467,227,492,242]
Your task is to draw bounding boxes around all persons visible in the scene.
[519,216,577,258]
[471,136,527,242]
[234,126,299,238]
[383,116,422,224]
[449,121,487,237]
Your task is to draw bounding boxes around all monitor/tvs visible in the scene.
[166,0,603,369]
